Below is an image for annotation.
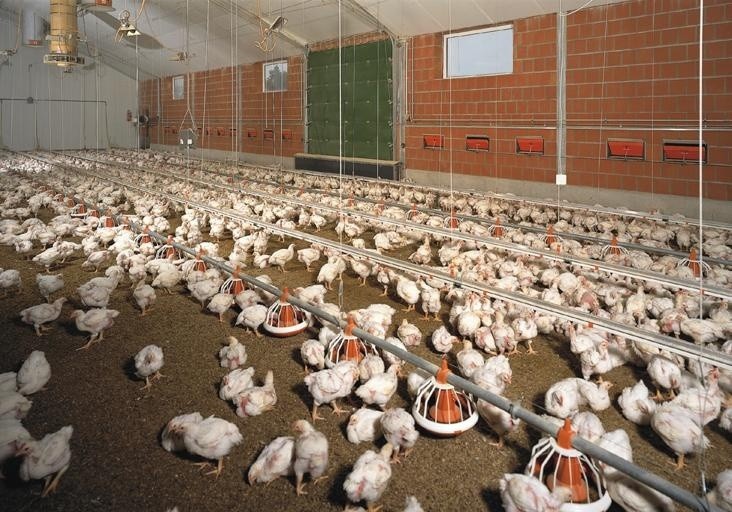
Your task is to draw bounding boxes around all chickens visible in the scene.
[0,147,732,512]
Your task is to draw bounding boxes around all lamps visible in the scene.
[256,0,288,52]
[167,0,192,63]
[118,0,141,37]
